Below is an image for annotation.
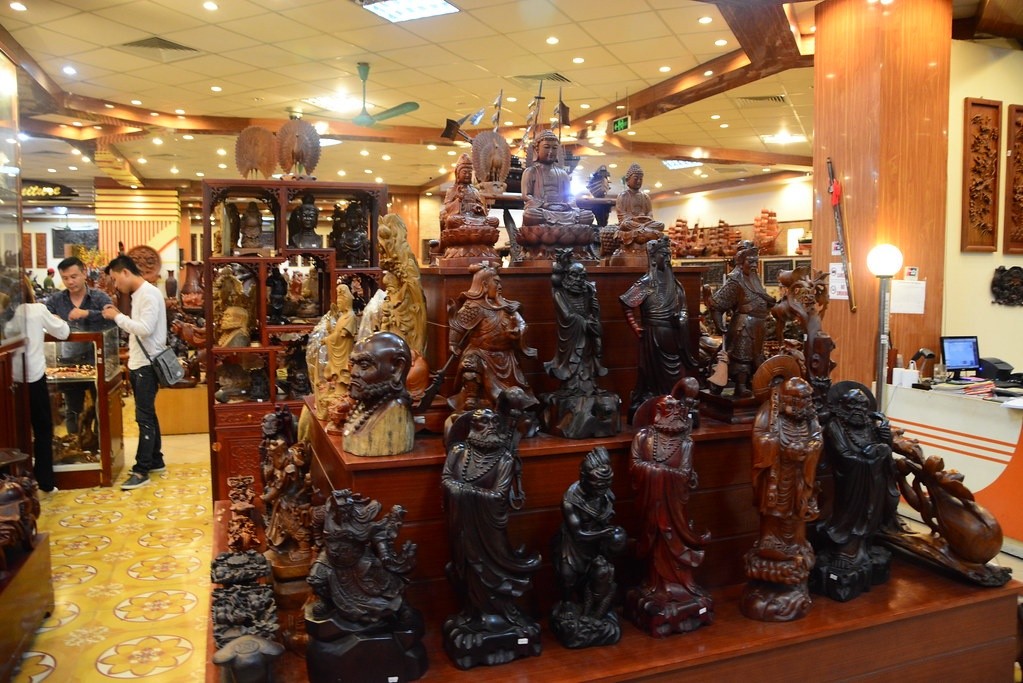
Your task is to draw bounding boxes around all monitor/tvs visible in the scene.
[940,336,981,381]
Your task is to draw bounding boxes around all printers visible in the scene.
[975,358,1014,381]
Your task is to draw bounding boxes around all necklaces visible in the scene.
[462,441,506,482]
[218,331,234,346]
[842,420,880,449]
[653,431,685,462]
[346,395,390,430]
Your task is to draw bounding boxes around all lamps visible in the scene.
[867,244,904,416]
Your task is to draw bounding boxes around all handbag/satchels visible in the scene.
[151,347,185,386]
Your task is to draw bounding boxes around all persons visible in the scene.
[627,191,644,215]
[45,256,112,455]
[44,268,55,289]
[4,276,71,494]
[102,255,167,489]
[211,132,904,683]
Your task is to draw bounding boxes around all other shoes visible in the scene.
[37,487,58,500]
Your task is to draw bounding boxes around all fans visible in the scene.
[286,63,420,131]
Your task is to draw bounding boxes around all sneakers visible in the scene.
[131,462,166,474]
[120,473,150,490]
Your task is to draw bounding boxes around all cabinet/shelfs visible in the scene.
[870,381,1023,560]
[198,177,388,513]
